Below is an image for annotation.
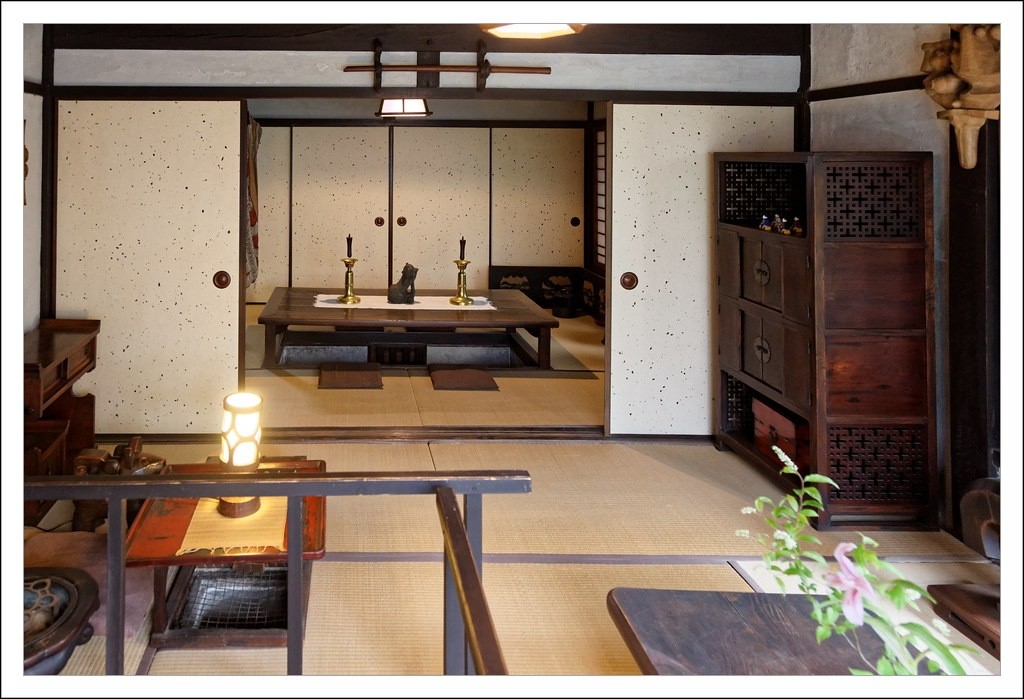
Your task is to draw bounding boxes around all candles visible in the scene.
[346,234,352,258]
[459,236,466,260]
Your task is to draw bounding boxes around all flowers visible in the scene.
[735,444,980,675]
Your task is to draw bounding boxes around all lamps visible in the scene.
[479,23,587,40]
[374,98,433,118]
[215,392,264,518]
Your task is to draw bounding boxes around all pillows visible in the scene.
[318,362,382,389]
[429,370,499,390]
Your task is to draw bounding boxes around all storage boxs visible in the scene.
[750,390,814,472]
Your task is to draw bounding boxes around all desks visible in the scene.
[122,459,328,650]
[257,287,559,371]
[606,587,951,675]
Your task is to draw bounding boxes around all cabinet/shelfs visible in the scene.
[713,147,943,531]
[23,317,102,529]
